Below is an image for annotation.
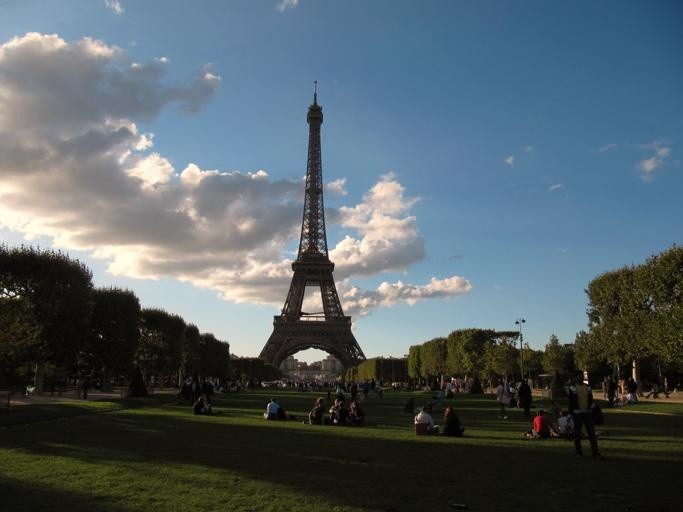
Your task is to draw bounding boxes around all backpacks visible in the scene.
[415,415,430,435]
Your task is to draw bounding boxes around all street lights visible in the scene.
[514,318,526,380]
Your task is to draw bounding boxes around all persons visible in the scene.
[493,368,669,459]
[260,372,383,428]
[391,369,490,437]
[46,365,257,416]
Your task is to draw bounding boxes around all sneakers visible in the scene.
[575,452,602,460]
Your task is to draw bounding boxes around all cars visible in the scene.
[261,379,290,391]
